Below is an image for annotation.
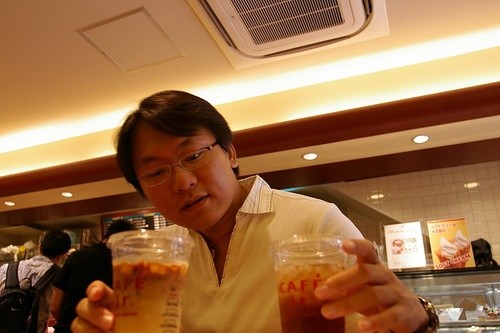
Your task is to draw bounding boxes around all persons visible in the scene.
[471,238,498,267]
[50,219,141,333]
[0,228,72,333]
[71,89,440,333]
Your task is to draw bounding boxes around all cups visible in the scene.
[268,234,348,333]
[107,230,192,333]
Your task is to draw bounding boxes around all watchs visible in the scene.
[389,295,440,333]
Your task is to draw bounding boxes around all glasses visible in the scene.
[138,140,218,187]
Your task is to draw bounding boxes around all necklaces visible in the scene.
[199,228,236,261]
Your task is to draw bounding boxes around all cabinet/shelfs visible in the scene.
[393,265,500,333]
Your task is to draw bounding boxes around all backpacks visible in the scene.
[0,261,61,333]
